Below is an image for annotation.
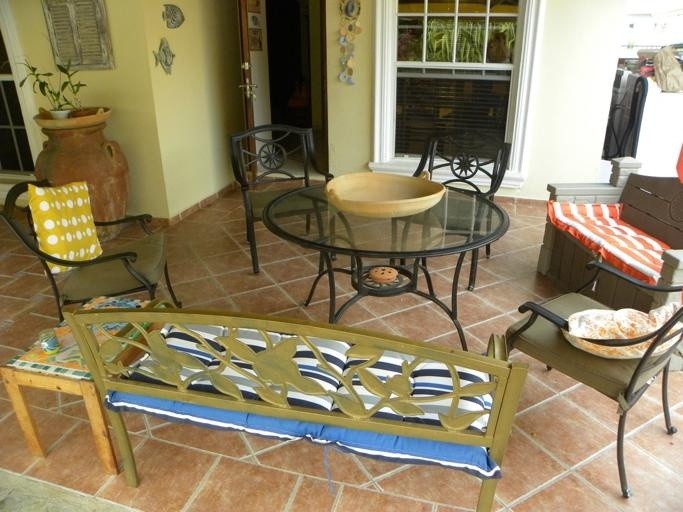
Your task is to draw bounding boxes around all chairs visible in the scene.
[389,130,512,291]
[0,177,183,322]
[505,259,683,499]
[230,124,337,274]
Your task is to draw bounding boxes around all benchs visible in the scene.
[60,301,531,512]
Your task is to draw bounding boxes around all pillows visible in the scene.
[27,180,104,281]
[560,301,683,360]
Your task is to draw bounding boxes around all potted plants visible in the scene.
[19,58,89,119]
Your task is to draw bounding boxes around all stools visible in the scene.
[1,295,178,476]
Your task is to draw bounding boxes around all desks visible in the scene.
[262,182,510,352]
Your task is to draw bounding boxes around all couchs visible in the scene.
[536,156,683,315]
